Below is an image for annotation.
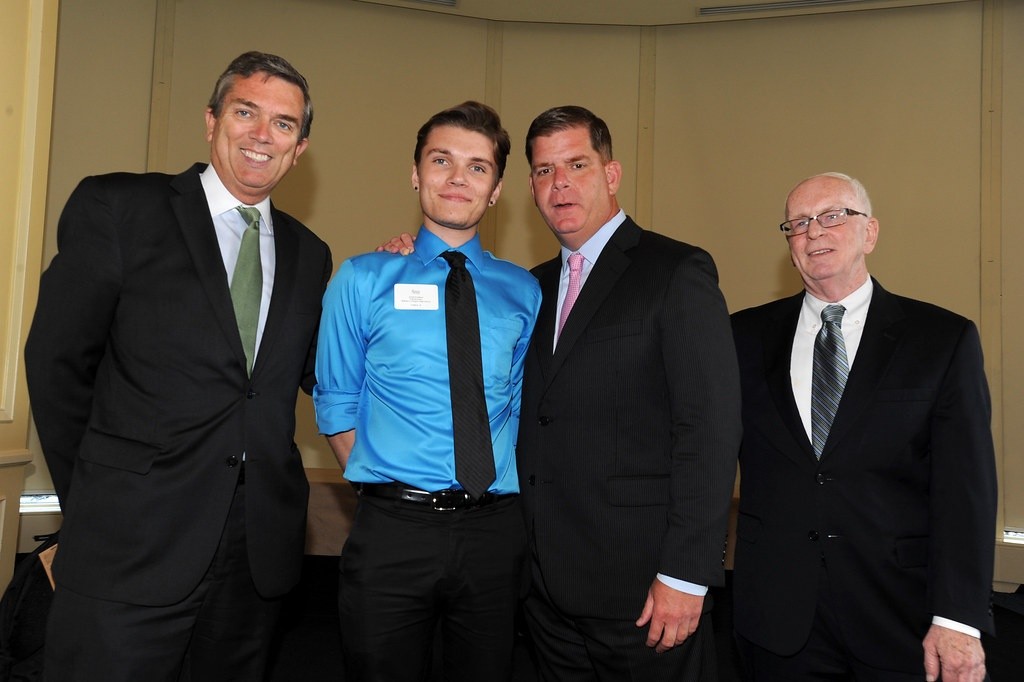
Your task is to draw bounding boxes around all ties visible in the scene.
[231,207,263,378]
[438,250,499,502]
[558,250,587,340]
[811,304,850,463]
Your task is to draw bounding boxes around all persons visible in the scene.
[732,171,1000,682]
[23,49,335,682]
[309,99,544,681]
[378,105,744,681]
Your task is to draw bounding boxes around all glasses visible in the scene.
[780,207,869,236]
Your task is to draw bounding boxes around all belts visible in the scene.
[362,481,518,513]
